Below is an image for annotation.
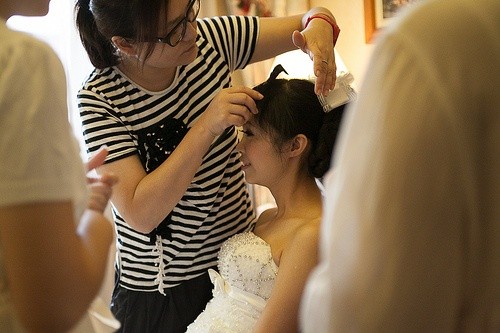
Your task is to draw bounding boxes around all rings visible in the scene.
[322,60,329,66]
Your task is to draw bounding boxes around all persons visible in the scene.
[297,0,500,333]
[184,72,356,333]
[0,0,120,333]
[74,0,341,332]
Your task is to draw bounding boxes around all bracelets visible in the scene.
[305,12,339,47]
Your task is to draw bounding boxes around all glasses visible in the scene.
[126,0,201,47]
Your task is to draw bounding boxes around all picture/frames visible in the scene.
[363,0,415,44]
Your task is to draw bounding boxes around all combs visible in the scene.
[248,64,291,109]
